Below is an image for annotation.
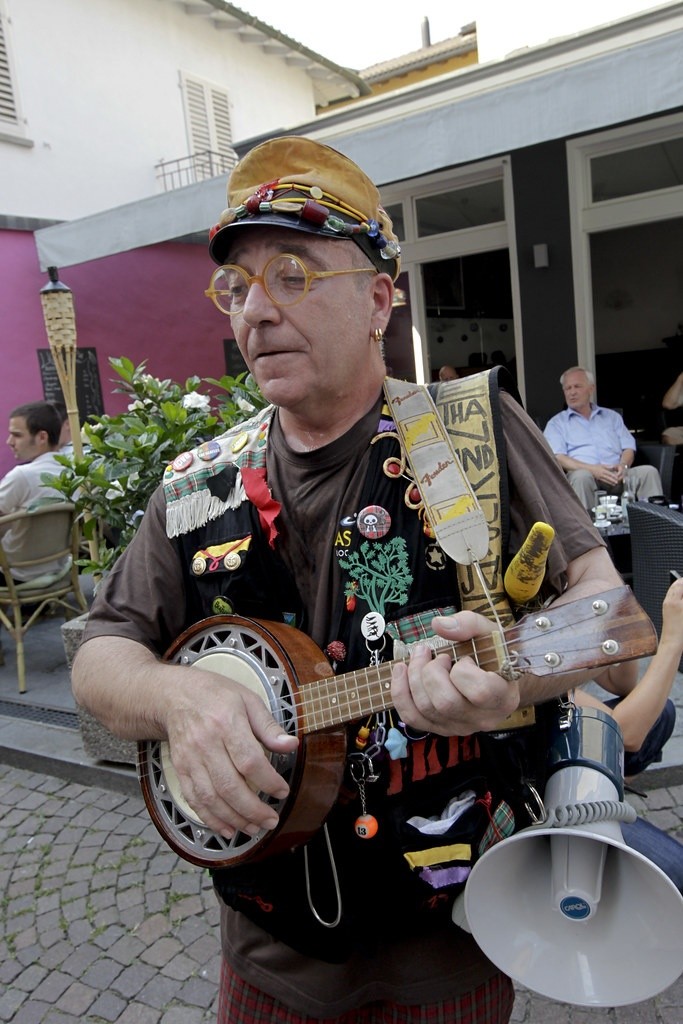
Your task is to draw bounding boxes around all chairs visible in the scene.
[0,503,89,693]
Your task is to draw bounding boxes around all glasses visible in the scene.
[202,254,380,307]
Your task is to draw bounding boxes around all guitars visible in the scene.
[132,586,657,873]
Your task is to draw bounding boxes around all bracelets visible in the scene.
[620,463,629,470]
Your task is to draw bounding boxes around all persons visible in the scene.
[0,400,81,625]
[440,366,458,383]
[544,367,665,518]
[69,137,632,1023]
[662,373,683,513]
[567,576,683,779]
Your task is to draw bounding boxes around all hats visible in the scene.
[207,136,403,282]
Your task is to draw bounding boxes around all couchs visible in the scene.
[635,443,675,503]
[627,503,683,643]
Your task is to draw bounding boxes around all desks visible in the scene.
[586,508,631,583]
